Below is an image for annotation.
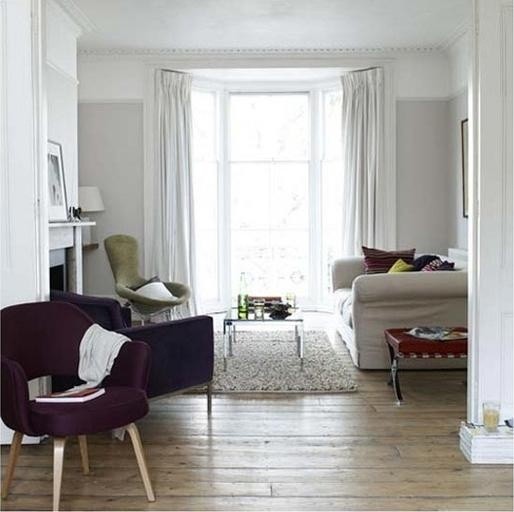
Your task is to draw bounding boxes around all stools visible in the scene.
[383,327,467,401]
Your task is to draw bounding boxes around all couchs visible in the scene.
[329,246,468,371]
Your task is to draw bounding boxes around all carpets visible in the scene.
[182,331,357,393]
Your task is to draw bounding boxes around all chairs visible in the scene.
[0,302,155,511]
[104,233,192,327]
[52,290,213,413]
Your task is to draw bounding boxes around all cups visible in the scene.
[251,297,265,319]
[481,401,502,431]
[283,286,295,311]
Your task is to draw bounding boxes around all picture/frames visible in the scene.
[48,140,69,223]
[461,119,468,218]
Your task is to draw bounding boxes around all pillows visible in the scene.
[361,245,455,274]
[132,276,176,301]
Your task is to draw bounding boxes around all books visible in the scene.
[34,388,106,403]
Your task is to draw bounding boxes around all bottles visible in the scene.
[236,272,249,318]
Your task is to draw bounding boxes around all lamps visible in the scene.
[78,186,104,244]
